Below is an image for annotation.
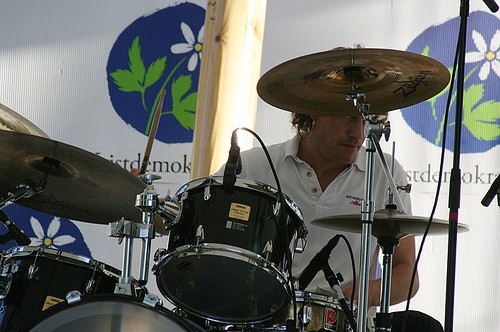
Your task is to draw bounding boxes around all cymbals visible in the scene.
[309,208,471,241]
[0,129,147,225]
[257,49,451,118]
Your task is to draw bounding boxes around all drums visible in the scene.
[151,176,305,326]
[0,245,149,331]
[256,290,359,332]
[14,290,206,332]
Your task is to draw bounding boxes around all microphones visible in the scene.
[230,131,242,175]
[295,235,341,291]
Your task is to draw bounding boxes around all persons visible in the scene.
[209,112,444,332]
